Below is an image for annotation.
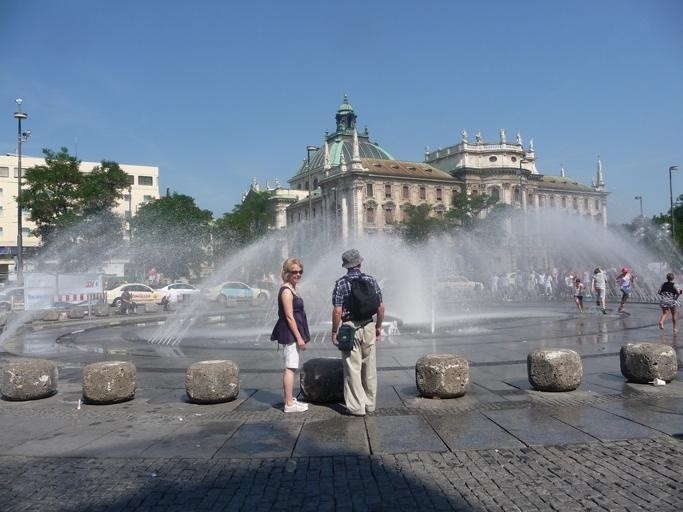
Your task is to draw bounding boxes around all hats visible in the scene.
[341,249,364,268]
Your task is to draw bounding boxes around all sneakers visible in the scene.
[284,397,308,412]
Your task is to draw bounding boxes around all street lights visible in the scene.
[518,156,535,208]
[668,165,679,239]
[306,146,320,220]
[12,97,32,310]
[634,197,643,216]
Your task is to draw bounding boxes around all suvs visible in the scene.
[436,273,483,301]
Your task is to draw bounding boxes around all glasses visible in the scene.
[289,270,303,274]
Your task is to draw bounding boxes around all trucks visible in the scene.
[0,272,103,312]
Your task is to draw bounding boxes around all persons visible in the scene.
[572,275,587,315]
[658,273,682,335]
[269,257,311,413]
[332,249,384,418]
[615,267,634,313]
[121,287,138,315]
[591,268,608,315]
[160,295,169,312]
[483,259,617,298]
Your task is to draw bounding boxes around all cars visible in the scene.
[204,281,271,302]
[154,283,201,302]
[104,283,170,307]
[498,270,538,293]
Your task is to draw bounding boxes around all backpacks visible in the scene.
[344,275,378,321]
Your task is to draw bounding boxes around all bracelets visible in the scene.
[332,330,337,335]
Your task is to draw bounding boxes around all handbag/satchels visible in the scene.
[337,325,355,351]
